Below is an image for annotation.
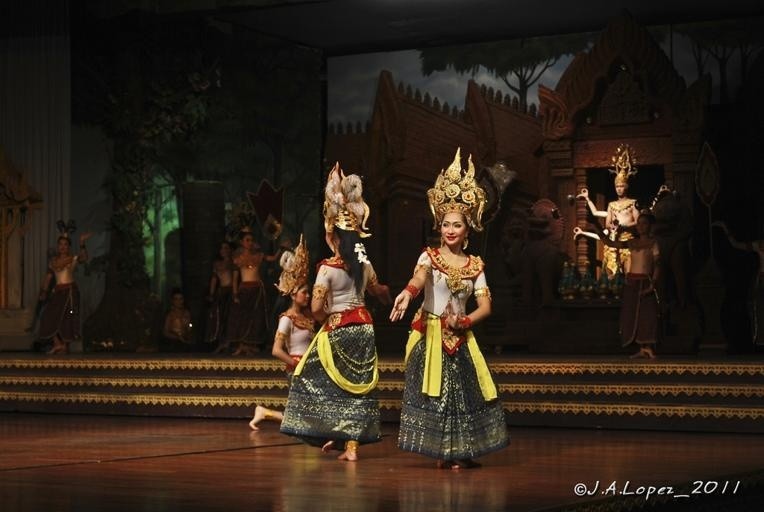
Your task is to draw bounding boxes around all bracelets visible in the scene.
[292,359,299,369]
[457,315,471,330]
[232,292,239,299]
[79,244,85,250]
[404,284,418,298]
[40,287,47,293]
[208,292,214,298]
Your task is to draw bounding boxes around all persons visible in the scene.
[278,223,394,461]
[224,224,290,356]
[248,282,317,430]
[161,283,214,354]
[208,241,241,355]
[37,218,94,356]
[592,213,664,361]
[389,211,511,470]
[572,183,670,277]
